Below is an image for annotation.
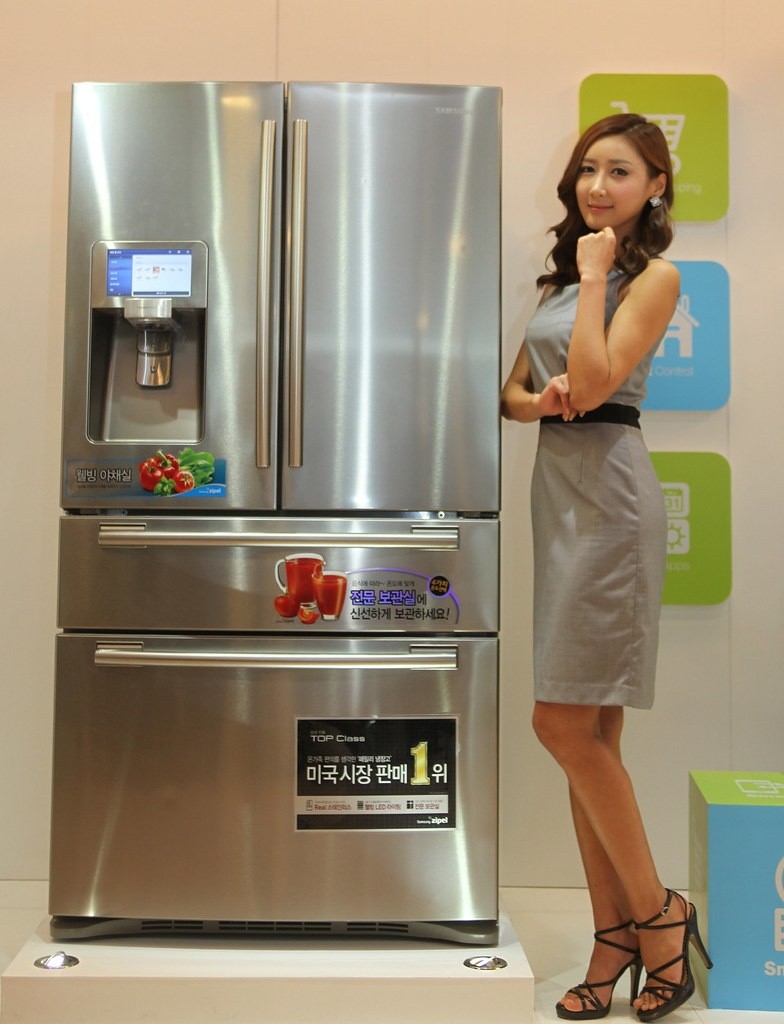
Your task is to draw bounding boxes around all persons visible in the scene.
[501,114,712,1023]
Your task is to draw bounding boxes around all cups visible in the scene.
[311,571,347,621]
[274,553,326,608]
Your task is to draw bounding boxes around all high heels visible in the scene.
[631,887,713,1021]
[556,920,643,1019]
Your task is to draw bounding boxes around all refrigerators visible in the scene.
[48,82,502,942]
